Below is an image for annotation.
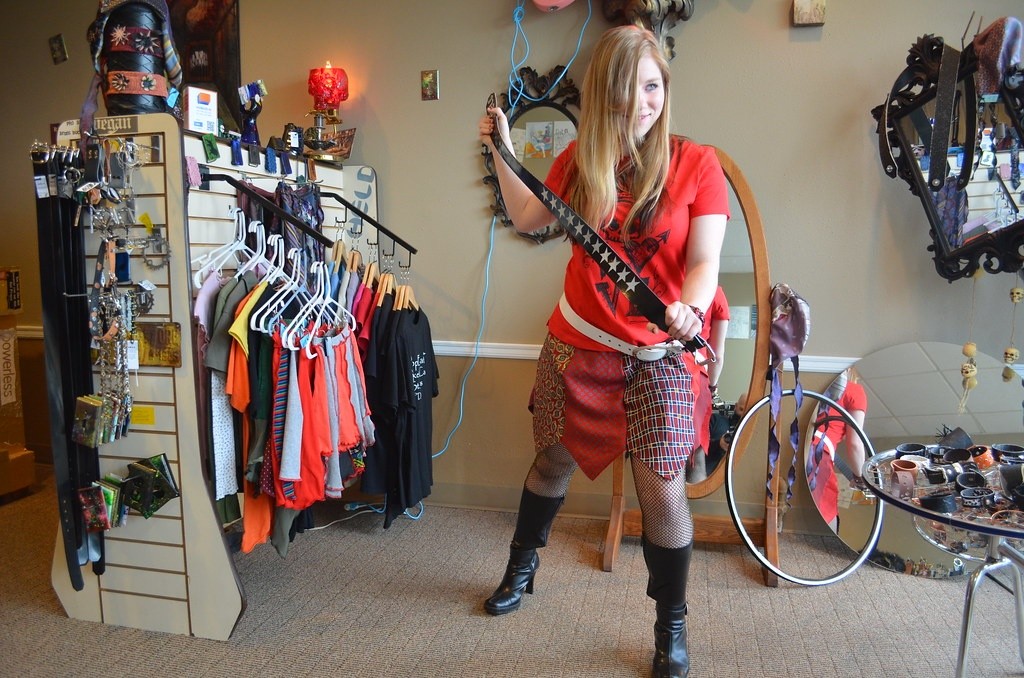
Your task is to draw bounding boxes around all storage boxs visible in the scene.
[182,86,218,136]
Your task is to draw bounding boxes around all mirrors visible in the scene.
[805,342,1024,584]
[480,65,582,245]
[602,143,779,588]
[872,31,1024,284]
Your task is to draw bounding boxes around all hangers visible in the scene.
[190,208,356,359]
[331,221,420,311]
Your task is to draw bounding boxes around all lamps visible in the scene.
[304,61,346,163]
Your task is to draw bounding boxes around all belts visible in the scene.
[89,240,121,343]
[29,142,108,589]
[558,292,685,363]
[877,44,981,190]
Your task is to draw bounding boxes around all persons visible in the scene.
[699,286,731,483]
[477,22,729,678]
[806,367,868,534]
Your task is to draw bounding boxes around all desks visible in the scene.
[911,513,1024,563]
[862,445,1024,678]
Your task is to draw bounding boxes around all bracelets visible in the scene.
[709,383,718,393]
[852,474,863,484]
[688,306,705,328]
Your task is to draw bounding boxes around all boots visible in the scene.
[485,481,568,615]
[638,529,692,678]
